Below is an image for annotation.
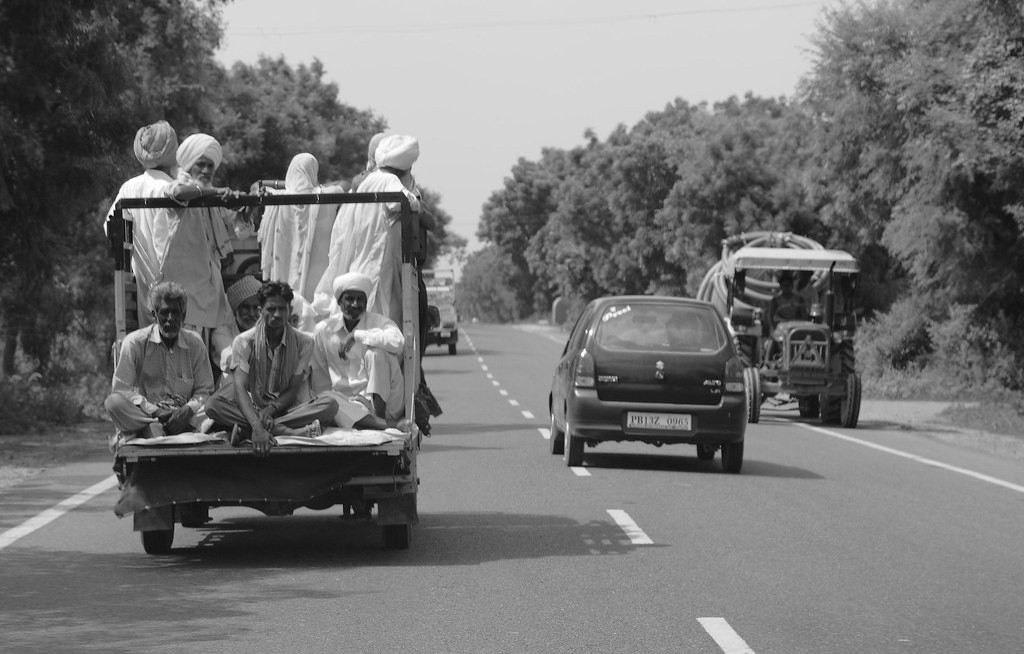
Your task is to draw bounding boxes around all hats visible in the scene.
[226,275,263,310]
[366,133,385,168]
[332,271,373,303]
[133,120,176,169]
[375,135,420,171]
[176,133,222,173]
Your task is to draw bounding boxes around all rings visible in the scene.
[268,420,271,422]
[257,448,260,451]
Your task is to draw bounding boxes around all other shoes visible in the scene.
[135,422,164,439]
[189,414,215,434]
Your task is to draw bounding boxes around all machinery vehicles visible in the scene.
[700,229,868,425]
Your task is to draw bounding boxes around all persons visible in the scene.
[760,271,820,371]
[103,121,435,456]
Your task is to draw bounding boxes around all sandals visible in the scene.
[303,420,322,437]
[230,423,251,445]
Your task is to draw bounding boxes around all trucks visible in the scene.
[108,178,425,553]
[422,268,459,356]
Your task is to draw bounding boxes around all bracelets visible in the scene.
[269,400,280,414]
[274,179,281,189]
[196,186,205,200]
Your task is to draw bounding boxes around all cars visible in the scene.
[546,292,748,472]
